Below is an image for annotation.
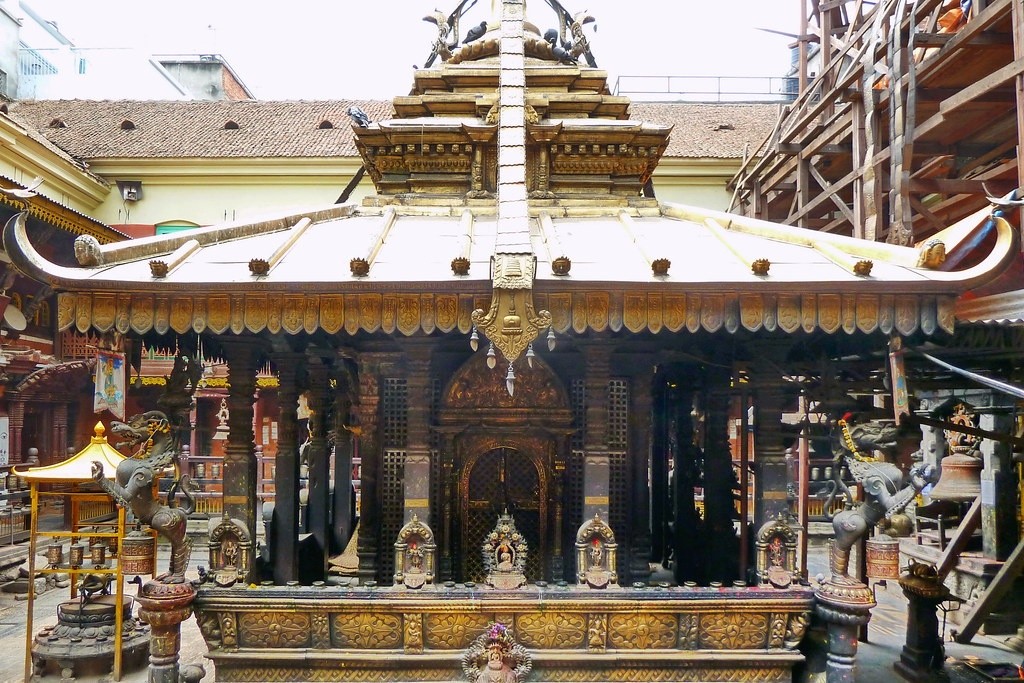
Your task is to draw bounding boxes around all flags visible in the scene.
[93,349,126,424]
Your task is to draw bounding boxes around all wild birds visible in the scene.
[461,21,488,45]
[345,106,373,128]
[543,28,579,66]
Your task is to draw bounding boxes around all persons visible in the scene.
[651,258,671,277]
[226,542,236,566]
[148,260,168,278]
[539,167,548,185]
[853,260,873,276]
[474,167,482,185]
[451,257,470,275]
[552,256,571,276]
[248,258,270,276]
[499,546,513,568]
[409,543,424,568]
[771,538,782,566]
[74,234,101,266]
[752,258,771,276]
[916,238,946,270]
[591,541,602,566]
[349,258,370,277]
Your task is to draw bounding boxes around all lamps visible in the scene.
[116,179,144,203]
